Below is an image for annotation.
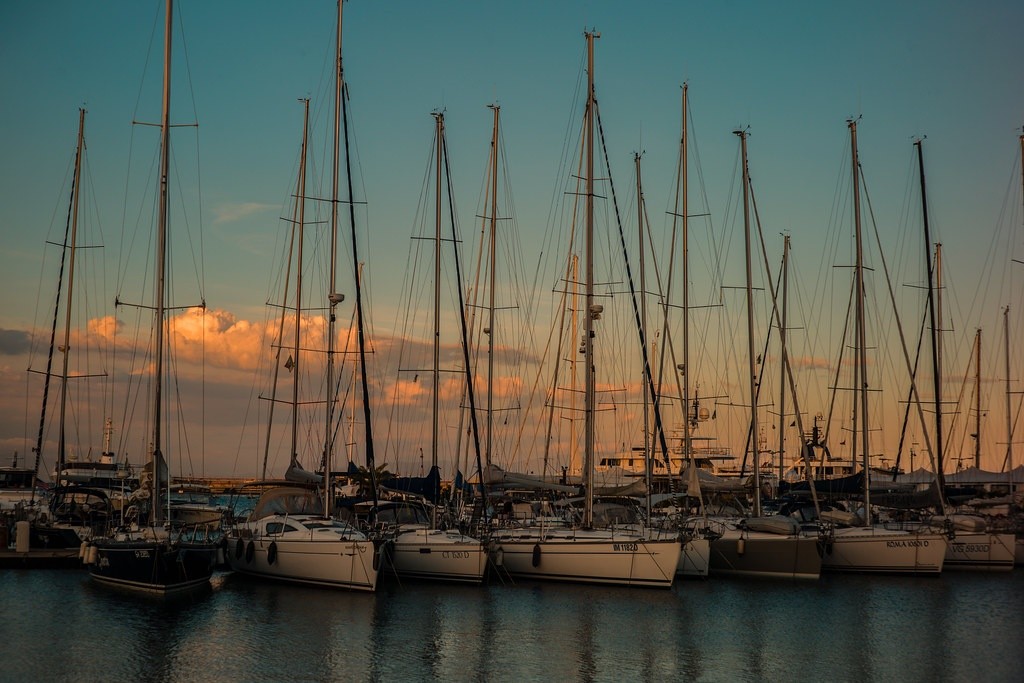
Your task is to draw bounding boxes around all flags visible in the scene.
[284,355,294,372]
[790,421,795,427]
[712,410,716,420]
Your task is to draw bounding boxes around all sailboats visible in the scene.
[0,1,1024,607]
